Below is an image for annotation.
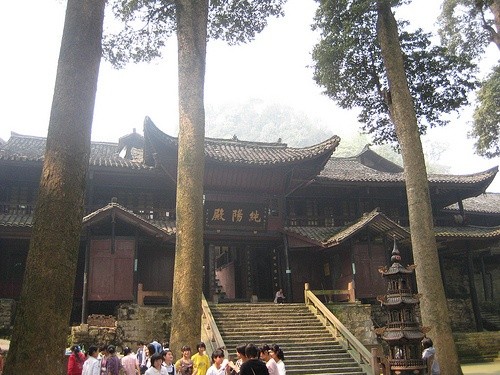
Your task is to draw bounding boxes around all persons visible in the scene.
[98,345,107,375]
[120,345,141,375]
[274,287,286,304]
[152,336,163,353]
[175,344,193,375]
[161,350,176,375]
[81,346,101,375]
[107,345,121,375]
[137,341,150,375]
[144,353,169,375]
[206,346,230,375]
[422,337,442,375]
[68,345,86,375]
[224,343,269,375]
[191,342,211,375]
[145,343,156,368]
[259,344,286,375]
